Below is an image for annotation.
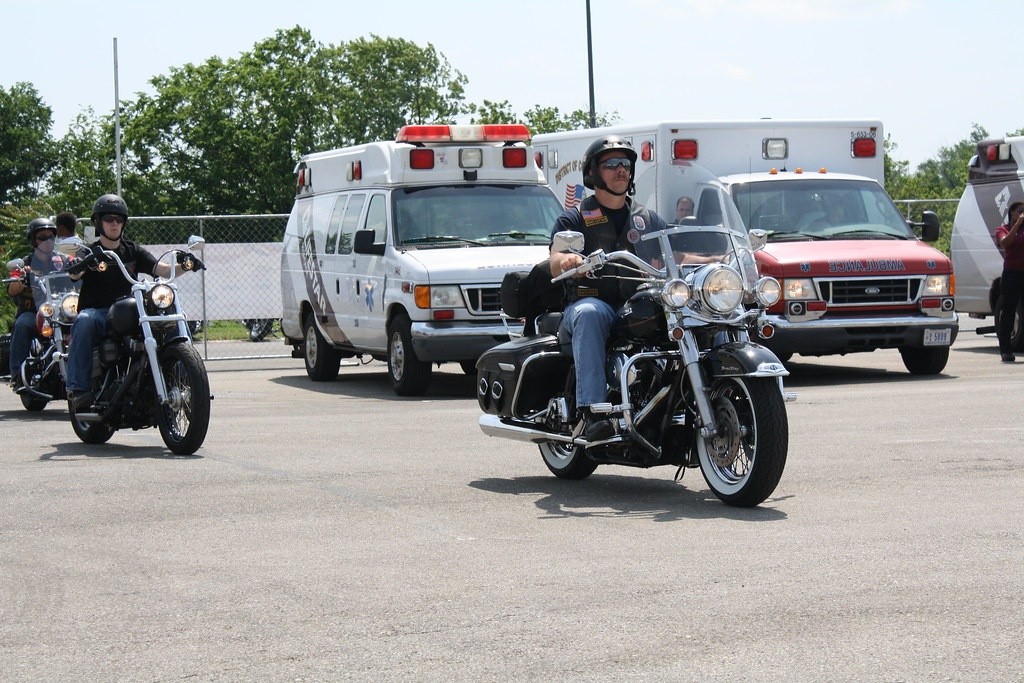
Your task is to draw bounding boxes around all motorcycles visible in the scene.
[475,159,788,509]
[55,236,213,456]
[2,236,89,411]
[242,319,278,341]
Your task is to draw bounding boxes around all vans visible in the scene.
[280,125,569,397]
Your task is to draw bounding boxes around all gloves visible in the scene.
[66,257,85,275]
[181,253,207,273]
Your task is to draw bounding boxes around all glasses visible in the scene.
[34,234,57,241]
[99,214,125,223]
[598,157,632,172]
[677,208,692,215]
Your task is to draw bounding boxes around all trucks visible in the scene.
[531,117,958,374]
[951,133,1024,352]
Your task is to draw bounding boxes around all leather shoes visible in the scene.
[584,410,615,442]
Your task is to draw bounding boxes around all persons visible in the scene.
[673,196,695,224]
[64,193,208,410]
[548,134,729,443]
[993,202,1024,362]
[7,217,71,392]
[54,213,84,255]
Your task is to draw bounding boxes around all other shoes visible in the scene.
[72,388,93,409]
[1000,352,1015,362]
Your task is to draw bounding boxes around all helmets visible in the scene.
[26,218,57,241]
[581,135,638,189]
[91,194,128,221]
